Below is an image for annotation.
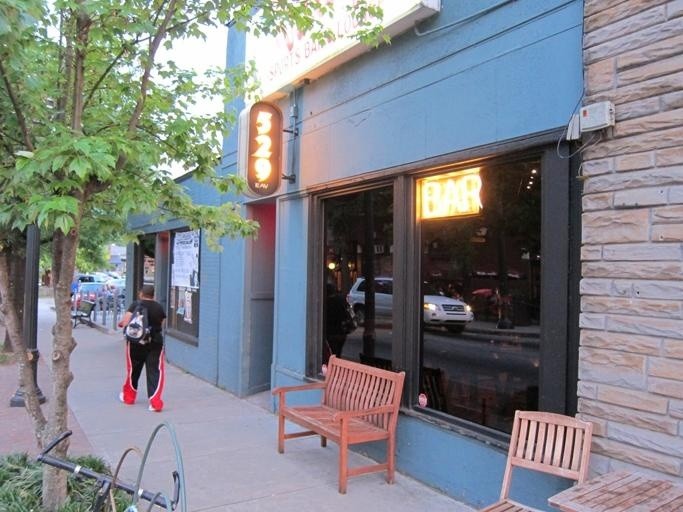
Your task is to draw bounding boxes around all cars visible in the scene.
[71,271,126,300]
[345,277,474,335]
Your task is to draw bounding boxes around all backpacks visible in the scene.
[122,299,151,345]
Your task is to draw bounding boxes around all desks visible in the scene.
[547,470,682,511]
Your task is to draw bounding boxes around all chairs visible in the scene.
[482,406,594,511]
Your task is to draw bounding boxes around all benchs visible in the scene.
[71,297,96,329]
[272,353,405,495]
[358,348,490,425]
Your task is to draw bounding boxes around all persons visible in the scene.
[118,285,166,412]
[323,280,357,364]
[497,285,513,320]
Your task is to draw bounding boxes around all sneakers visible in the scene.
[119,392,124,403]
[148,402,162,411]
